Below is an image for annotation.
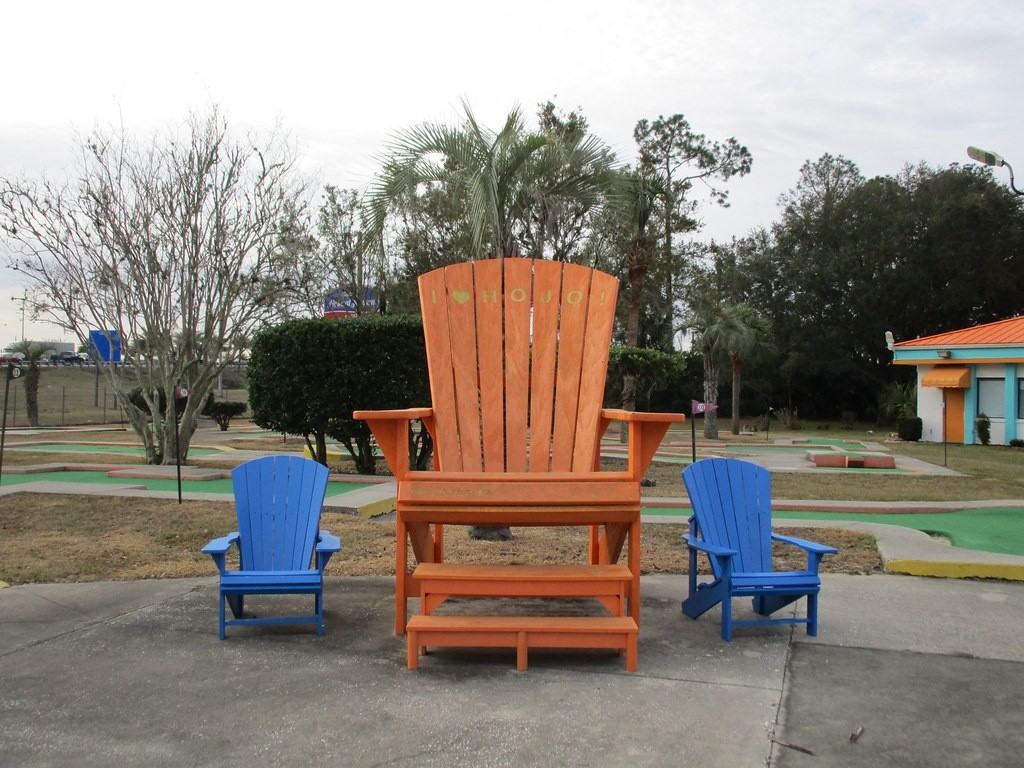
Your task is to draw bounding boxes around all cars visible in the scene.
[78,353,95,365]
[0,353,23,363]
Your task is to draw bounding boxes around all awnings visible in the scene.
[920,365,971,388]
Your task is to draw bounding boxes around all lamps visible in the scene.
[937,351,951,357]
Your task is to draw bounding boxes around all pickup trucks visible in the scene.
[50,351,85,366]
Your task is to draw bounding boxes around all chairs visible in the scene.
[680,457,839,643]
[354,257,686,671]
[200,455,342,641]
